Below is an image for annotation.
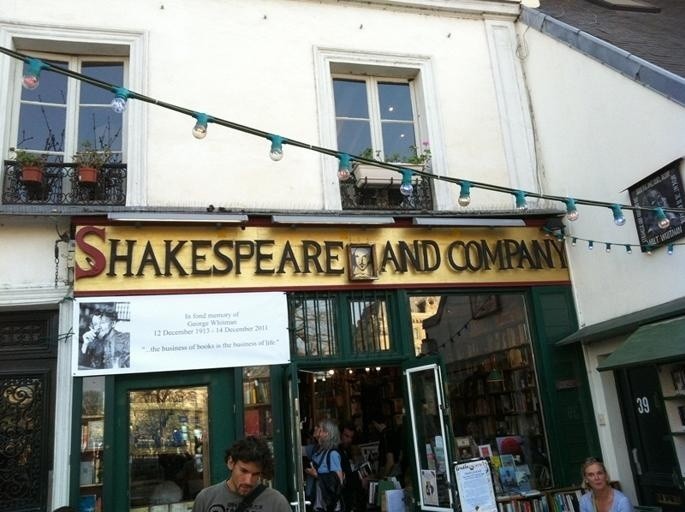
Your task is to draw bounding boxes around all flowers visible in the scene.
[349,136,432,165]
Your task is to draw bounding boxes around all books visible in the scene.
[441,322,553,498]
[496,487,591,512]
[241,380,271,404]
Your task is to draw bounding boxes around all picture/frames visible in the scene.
[346,241,380,282]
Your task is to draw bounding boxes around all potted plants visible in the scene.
[12,139,114,187]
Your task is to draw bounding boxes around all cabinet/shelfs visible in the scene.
[298,342,553,497]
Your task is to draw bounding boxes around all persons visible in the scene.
[578,456,635,512]
[190,435,295,512]
[300,409,411,512]
[350,246,375,278]
[77,306,130,370]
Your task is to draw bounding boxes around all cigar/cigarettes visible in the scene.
[88,326,94,332]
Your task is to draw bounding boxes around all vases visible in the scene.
[350,163,431,190]
